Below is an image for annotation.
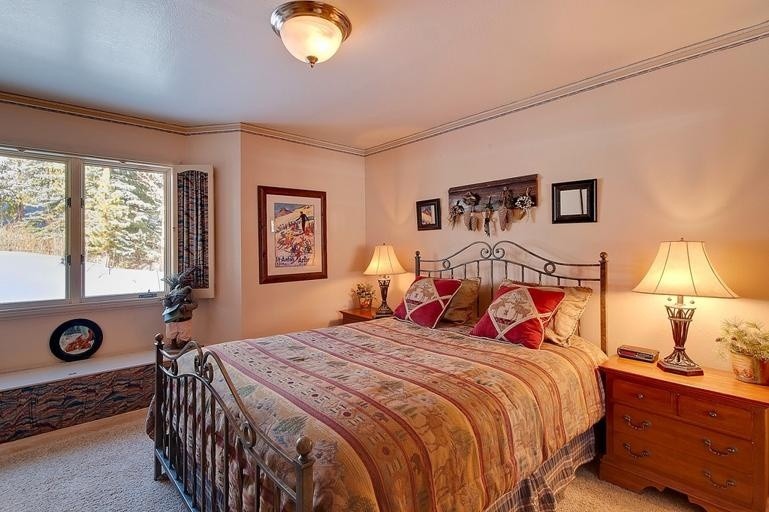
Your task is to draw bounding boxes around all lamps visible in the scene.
[631,237,740,376]
[363,243,407,318]
[271,0,352,67]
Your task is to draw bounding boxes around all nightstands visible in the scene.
[339,307,378,324]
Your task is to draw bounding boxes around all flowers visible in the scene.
[350,282,385,310]
[718,316,769,362]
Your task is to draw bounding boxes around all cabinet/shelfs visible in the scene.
[598,354,769,512]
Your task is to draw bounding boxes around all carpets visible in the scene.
[0,408,702,512]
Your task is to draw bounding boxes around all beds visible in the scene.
[155,241,607,512]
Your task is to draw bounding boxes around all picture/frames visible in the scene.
[257,186,328,284]
[49,318,103,361]
[552,179,597,223]
[416,198,441,230]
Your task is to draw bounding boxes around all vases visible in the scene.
[359,298,372,308]
[732,352,769,385]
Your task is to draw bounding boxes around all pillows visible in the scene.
[499,280,592,348]
[470,286,565,349]
[442,277,481,325]
[392,277,462,329]
